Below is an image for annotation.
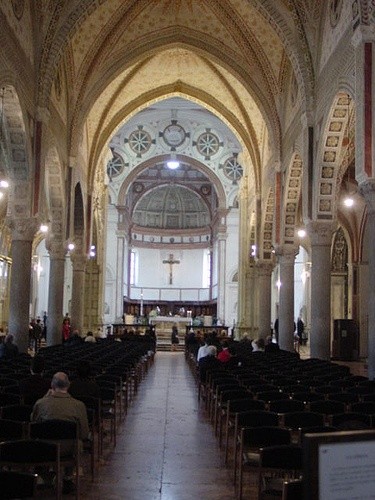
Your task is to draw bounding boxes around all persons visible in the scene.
[30,371,90,496]
[0,304,308,410]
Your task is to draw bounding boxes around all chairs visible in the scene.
[0,340,375,500]
